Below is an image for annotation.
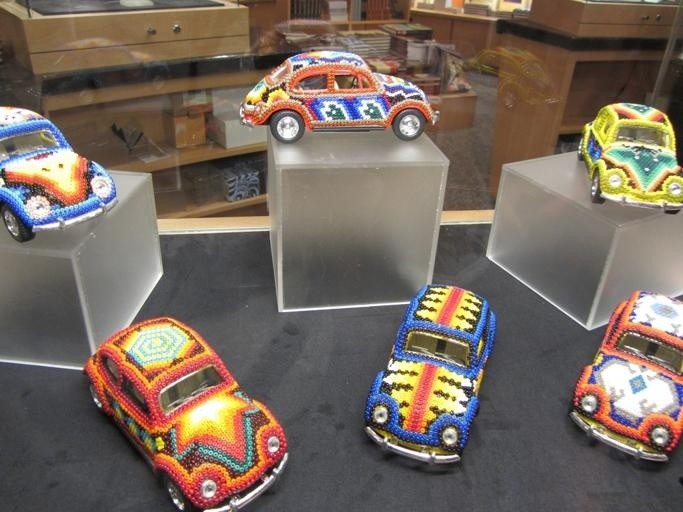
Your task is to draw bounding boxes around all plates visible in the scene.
[1,53,308,217]
[488,33,680,200]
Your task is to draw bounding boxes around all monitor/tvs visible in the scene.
[331,23,441,98]
